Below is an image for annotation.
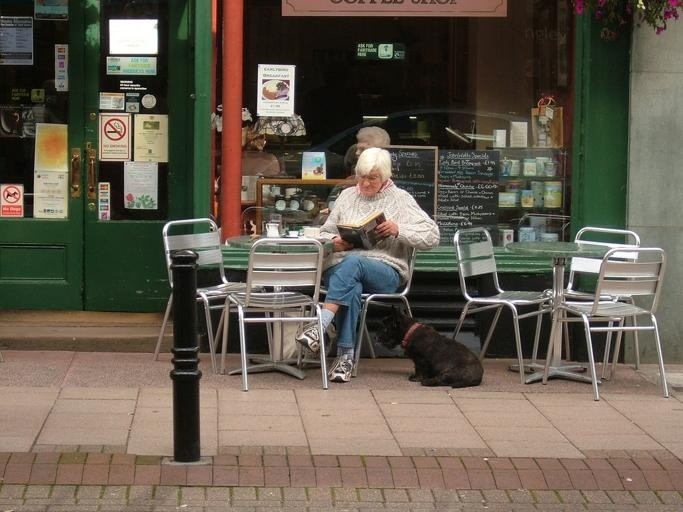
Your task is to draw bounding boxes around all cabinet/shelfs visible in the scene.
[487,145,567,241]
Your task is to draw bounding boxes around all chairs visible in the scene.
[220,237,327,391]
[319,246,418,376]
[452,225,669,402]
[154,217,273,374]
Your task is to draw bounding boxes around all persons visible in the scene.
[355,126,392,160]
[312,144,359,229]
[294,147,442,383]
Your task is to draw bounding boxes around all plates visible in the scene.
[262,80,289,101]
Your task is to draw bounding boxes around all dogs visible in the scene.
[375,303,485,389]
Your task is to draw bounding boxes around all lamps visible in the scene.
[259,116,307,179]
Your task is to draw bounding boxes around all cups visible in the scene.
[272,187,280,195]
[288,231,298,237]
[304,227,320,238]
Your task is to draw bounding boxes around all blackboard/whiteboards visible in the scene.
[381,145,500,245]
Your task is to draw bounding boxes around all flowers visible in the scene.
[567,0,683,43]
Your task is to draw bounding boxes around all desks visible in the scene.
[228,234,333,380]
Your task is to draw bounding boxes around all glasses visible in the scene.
[358,177,379,183]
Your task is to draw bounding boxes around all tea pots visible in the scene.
[266,224,280,237]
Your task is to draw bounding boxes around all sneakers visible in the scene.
[328,355,354,382]
[296,321,321,352]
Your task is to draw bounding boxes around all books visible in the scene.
[335,210,390,251]
